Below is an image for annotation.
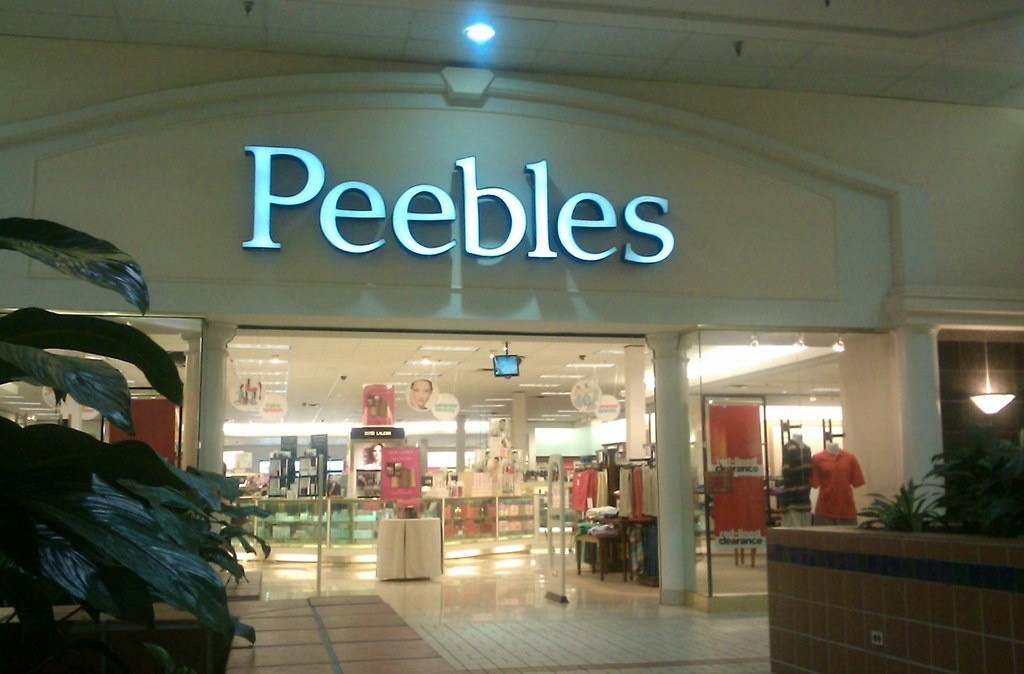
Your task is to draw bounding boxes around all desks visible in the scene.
[378,517,442,579]
[574,516,657,581]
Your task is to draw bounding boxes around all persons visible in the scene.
[489,419,519,474]
[327,443,385,497]
[410,381,432,410]
[782,433,866,526]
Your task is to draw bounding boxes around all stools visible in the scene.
[577,533,634,581]
